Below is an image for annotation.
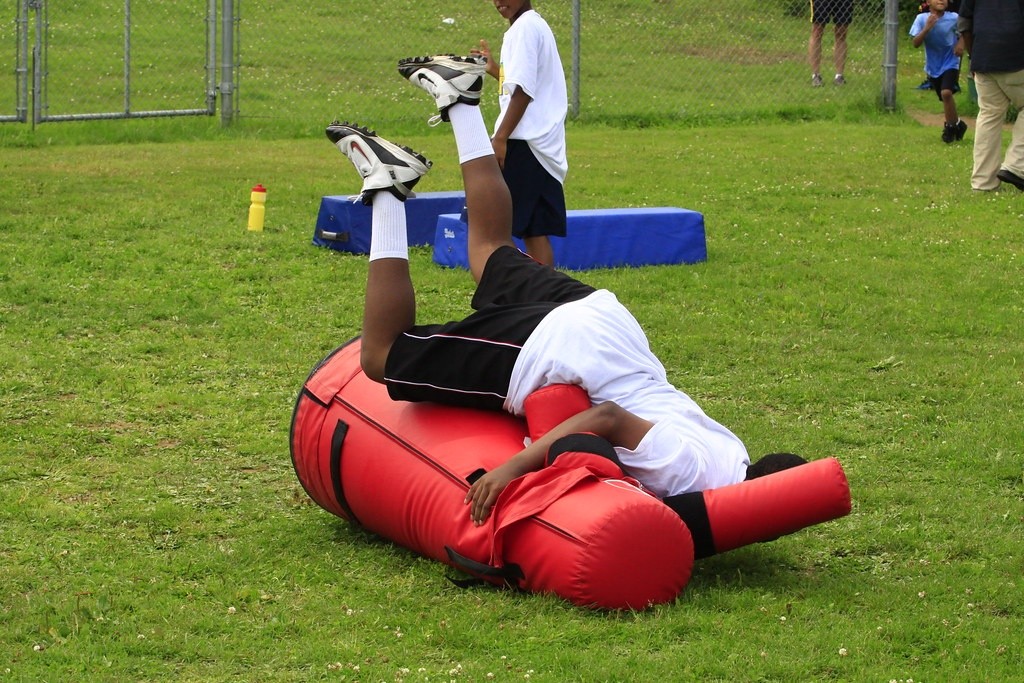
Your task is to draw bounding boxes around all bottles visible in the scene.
[246,182,267,231]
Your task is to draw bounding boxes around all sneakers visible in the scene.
[951,118,967,141]
[832,73,846,85]
[324,119,434,208]
[810,71,822,88]
[397,52,489,122]
[941,121,956,143]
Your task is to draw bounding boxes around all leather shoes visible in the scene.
[997,170,1024,192]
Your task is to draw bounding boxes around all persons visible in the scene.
[323,51,812,529]
[805,0,854,88]
[459,0,571,272]
[909,0,969,142]
[957,0,1024,190]
[909,0,965,93]
